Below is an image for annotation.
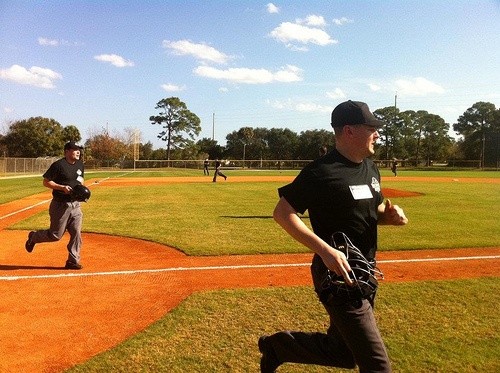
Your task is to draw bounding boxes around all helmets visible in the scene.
[72,185,91,202]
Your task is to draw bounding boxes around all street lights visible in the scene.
[243,144,247,160]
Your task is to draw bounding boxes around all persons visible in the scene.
[319,146,327,158]
[258,99,408,373]
[203,158,209,175]
[392,158,399,177]
[25,142,85,269]
[212,159,227,182]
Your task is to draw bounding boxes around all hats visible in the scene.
[331,100,386,126]
[64,142,83,150]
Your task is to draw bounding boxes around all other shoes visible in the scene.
[258,335,283,373]
[25,231,36,253]
[65,264,83,270]
